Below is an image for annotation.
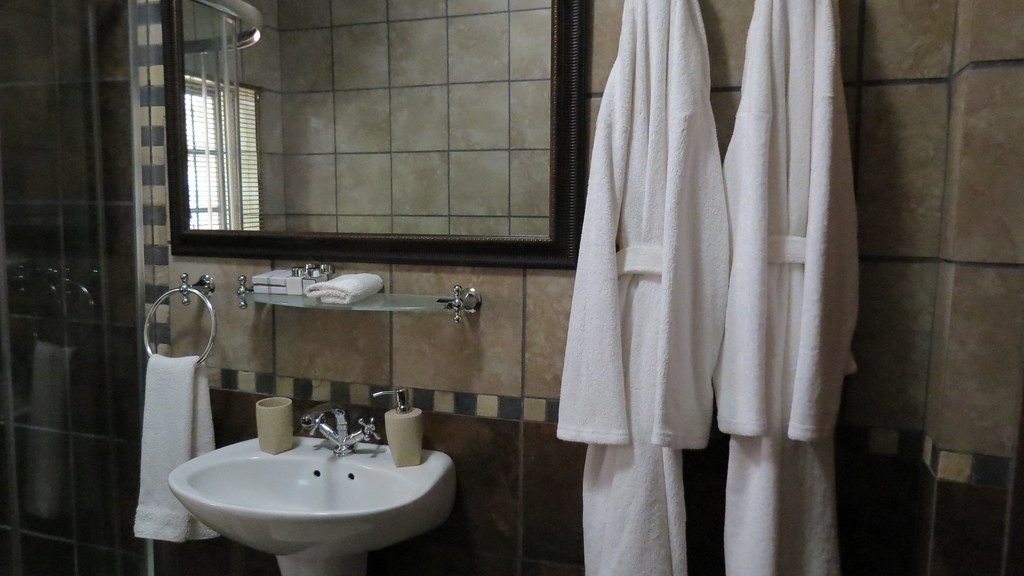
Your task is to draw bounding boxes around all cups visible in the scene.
[256,397,294,455]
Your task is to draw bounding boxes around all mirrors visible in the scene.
[161,0,586,267]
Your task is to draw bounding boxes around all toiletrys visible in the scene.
[372,387,424,468]
[286,262,335,295]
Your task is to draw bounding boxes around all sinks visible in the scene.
[166,436,455,558]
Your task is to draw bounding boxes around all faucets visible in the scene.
[300,400,382,456]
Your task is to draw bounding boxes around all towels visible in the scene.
[304,273,384,304]
[133,353,223,543]
[21,341,101,520]
